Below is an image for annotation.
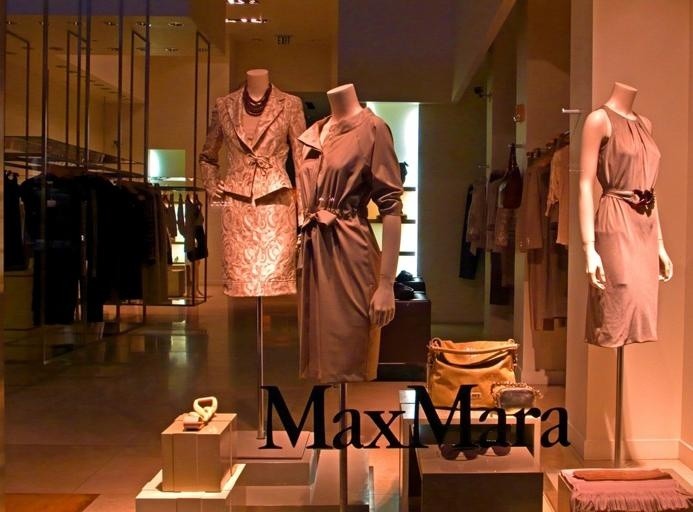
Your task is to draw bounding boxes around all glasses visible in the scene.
[439,441,480,461]
[473,439,512,456]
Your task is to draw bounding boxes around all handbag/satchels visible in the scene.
[497,143,522,210]
[426,337,521,412]
[490,381,544,411]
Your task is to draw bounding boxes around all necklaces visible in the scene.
[243,86,270,116]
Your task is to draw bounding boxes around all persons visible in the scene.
[298,82,403,385]
[578,80,674,349]
[199,69,307,299]
[187,193,203,298]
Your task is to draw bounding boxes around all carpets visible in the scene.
[0,491,100,511]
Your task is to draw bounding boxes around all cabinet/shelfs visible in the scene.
[369,186,419,257]
